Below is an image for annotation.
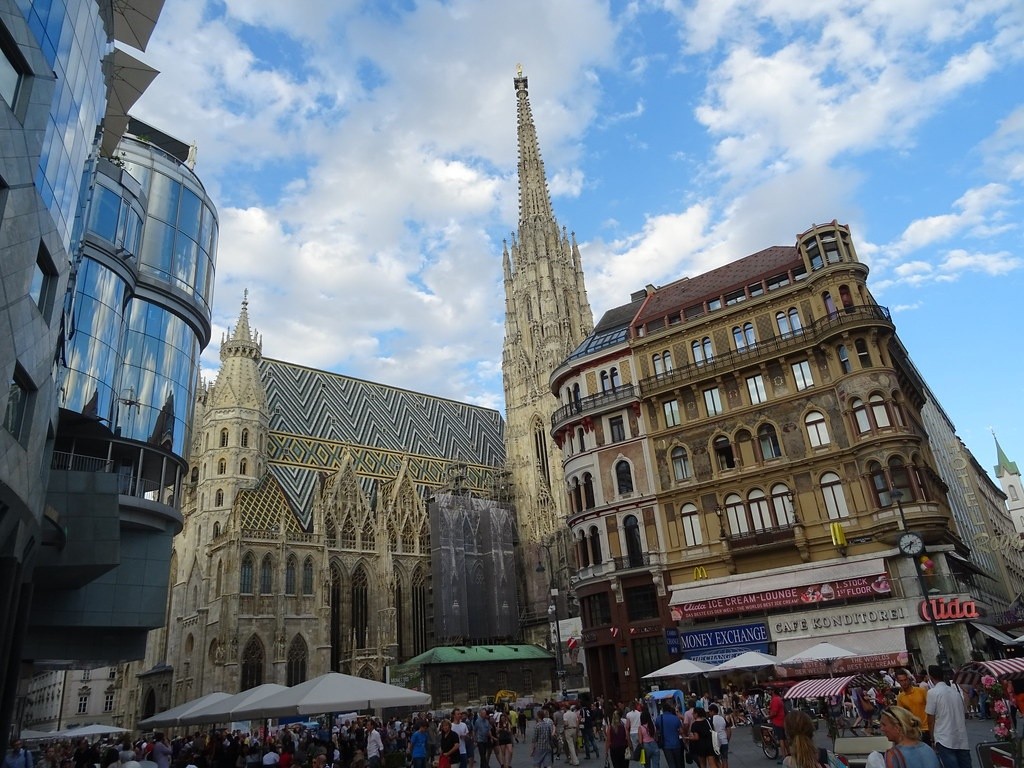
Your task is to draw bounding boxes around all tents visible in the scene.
[403,644,557,666]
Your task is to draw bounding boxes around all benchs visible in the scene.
[851,687,875,720]
[834,735,895,768]
[991,746,1015,768]
[976,742,1017,768]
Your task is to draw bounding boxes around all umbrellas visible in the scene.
[233,671,432,720]
[640,657,729,679]
[715,650,796,671]
[784,640,857,665]
[141,693,230,722]
[62,724,132,738]
[182,683,288,723]
[19,723,57,740]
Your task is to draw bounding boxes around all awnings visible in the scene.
[983,657,1024,675]
[785,675,855,698]
[970,621,1018,645]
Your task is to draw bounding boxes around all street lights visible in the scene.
[536,544,568,701]
[888,481,953,676]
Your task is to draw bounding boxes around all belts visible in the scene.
[566,727,577,729]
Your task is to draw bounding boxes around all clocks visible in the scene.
[898,532,925,557]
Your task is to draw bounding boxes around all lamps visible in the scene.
[714,505,724,516]
[787,492,794,502]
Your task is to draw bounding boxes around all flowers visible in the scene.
[981,674,1013,741]
[876,680,890,692]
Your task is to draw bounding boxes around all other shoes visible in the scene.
[978,718,984,721]
[515,737,519,743]
[731,725,736,728]
[568,756,570,758]
[584,757,590,759]
[777,756,785,764]
[1009,728,1018,733]
[467,760,476,763]
[573,764,579,766]
[500,764,504,768]
[554,757,560,760]
[596,751,599,758]
[973,715,979,718]
[508,766,512,768]
[569,764,572,765]
[969,716,972,720]
[849,728,858,736]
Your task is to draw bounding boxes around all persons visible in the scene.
[439,719,461,768]
[473,708,492,768]
[562,704,580,765]
[708,704,731,768]
[654,704,686,768]
[563,646,584,688]
[924,665,973,768]
[41,668,1024,768]
[604,710,634,768]
[638,712,660,767]
[879,705,942,768]
[531,711,556,768]
[894,669,935,749]
[781,710,831,768]
[366,720,385,768]
[406,720,431,768]
[578,702,599,760]
[684,707,716,768]
[762,685,792,765]
[498,713,515,768]
[451,709,469,768]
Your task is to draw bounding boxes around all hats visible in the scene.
[766,685,774,690]
[562,701,569,708]
[570,705,575,710]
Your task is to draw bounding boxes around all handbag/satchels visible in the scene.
[632,743,642,761]
[548,727,559,748]
[624,746,634,760]
[705,718,722,756]
[640,749,646,765]
[656,714,664,749]
[599,759,610,768]
[686,752,693,764]
